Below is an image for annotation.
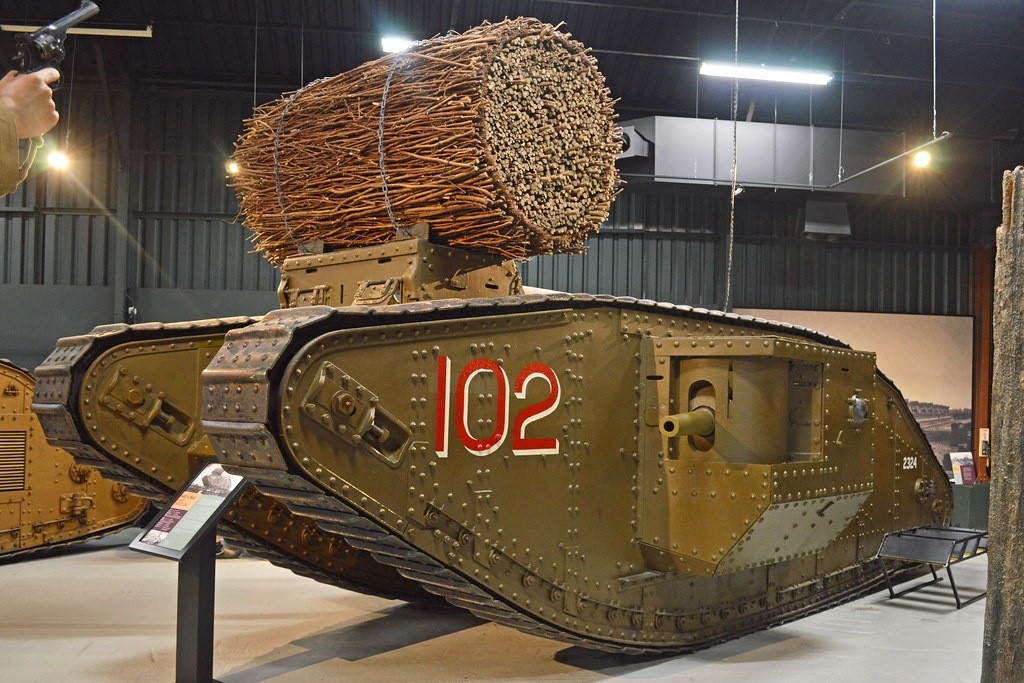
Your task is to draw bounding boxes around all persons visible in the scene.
[0,68,60,192]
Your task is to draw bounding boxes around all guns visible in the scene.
[10,0,106,91]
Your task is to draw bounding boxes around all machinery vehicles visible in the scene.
[31,237,952,654]
